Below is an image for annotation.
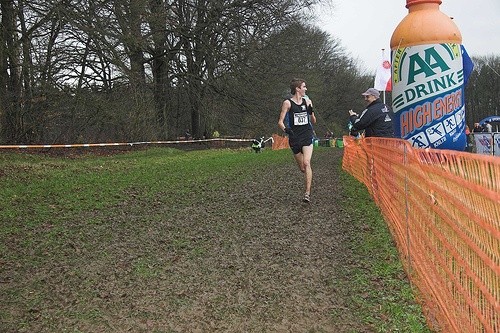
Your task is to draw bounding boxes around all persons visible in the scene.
[464,121,492,148]
[279,78,316,201]
[347,88,394,137]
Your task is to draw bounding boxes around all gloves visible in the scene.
[308,104,313,114]
[284,126,294,136]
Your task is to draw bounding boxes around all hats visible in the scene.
[361,88,380,98]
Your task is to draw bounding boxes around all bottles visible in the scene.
[389,0,467,152]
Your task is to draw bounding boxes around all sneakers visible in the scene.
[302,194,310,201]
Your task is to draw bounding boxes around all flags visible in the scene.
[375,56,393,91]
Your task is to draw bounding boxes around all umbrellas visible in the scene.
[480,116,500,131]
